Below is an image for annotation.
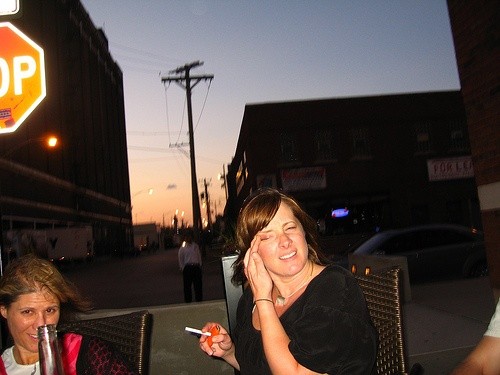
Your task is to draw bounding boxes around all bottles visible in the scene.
[38,324,62,375]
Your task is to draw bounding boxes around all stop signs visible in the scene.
[0,20,47,135]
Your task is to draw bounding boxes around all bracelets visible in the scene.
[254,298,274,304]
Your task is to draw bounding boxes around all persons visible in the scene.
[451,299,500,375]
[0,253,139,375]
[115,231,203,303]
[198,188,380,375]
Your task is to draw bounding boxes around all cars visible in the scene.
[353,224,486,282]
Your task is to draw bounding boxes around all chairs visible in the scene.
[357,265,409,375]
[56,310,149,375]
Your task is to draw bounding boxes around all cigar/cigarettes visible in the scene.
[185,326,212,337]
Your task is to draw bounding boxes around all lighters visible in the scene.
[207,325,220,347]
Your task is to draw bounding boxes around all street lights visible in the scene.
[0,136,58,164]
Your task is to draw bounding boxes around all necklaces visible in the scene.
[274,260,314,306]
